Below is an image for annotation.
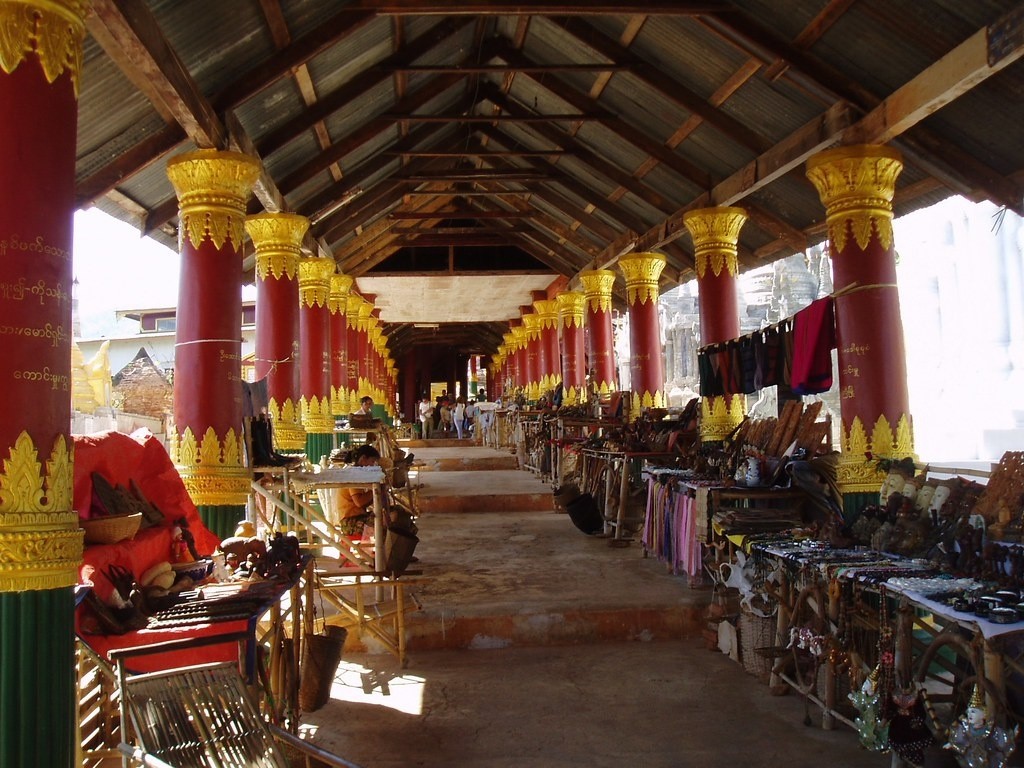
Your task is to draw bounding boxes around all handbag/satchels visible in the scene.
[392,460,407,488]
[554,483,580,511]
[299,624,348,712]
[565,493,604,536]
[383,524,420,579]
[391,447,406,463]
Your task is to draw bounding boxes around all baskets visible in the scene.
[817,665,850,704]
[80,512,143,544]
[740,609,776,679]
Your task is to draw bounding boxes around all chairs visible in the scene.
[107,631,363,768]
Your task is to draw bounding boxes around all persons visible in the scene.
[852,458,959,550]
[418,389,488,440]
[336,444,419,564]
[354,396,375,415]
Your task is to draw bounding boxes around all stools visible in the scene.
[340,536,371,568]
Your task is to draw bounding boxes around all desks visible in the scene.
[71,406,1024,768]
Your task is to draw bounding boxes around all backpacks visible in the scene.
[383,505,418,535]
